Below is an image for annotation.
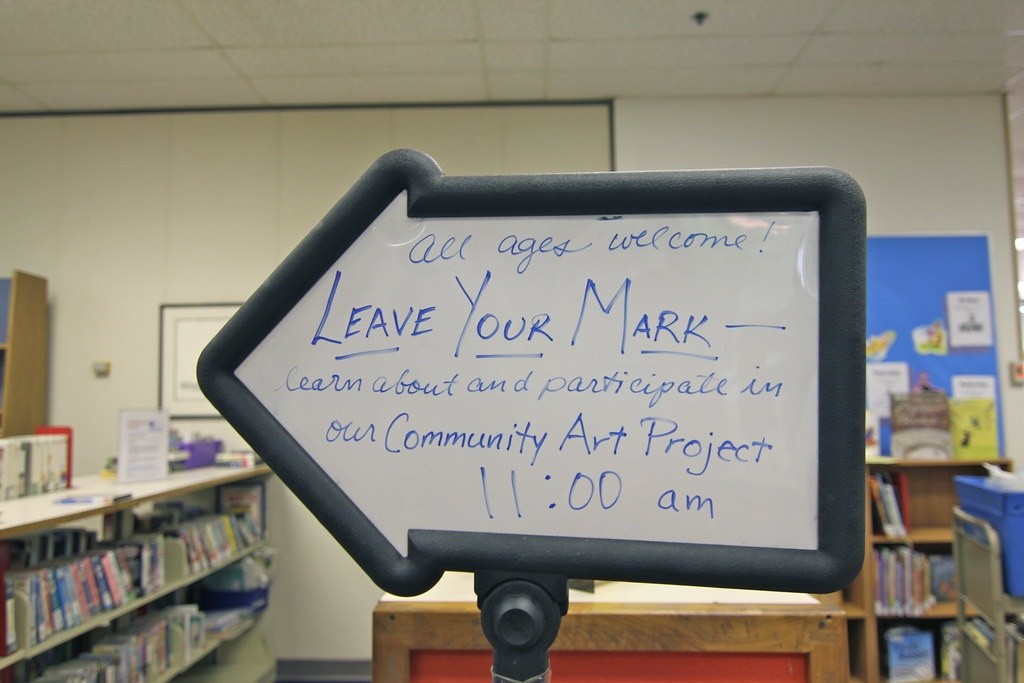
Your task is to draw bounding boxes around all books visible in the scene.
[0,432,259,683]
[867,387,961,683]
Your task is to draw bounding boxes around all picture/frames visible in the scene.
[157,301,241,419]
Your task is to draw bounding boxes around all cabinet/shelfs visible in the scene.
[0,465,274,682]
[843,453,1023,682]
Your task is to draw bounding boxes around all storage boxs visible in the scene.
[954,472,1024,601]
[885,628,936,683]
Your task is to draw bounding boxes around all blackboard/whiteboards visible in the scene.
[197,150,867,596]
